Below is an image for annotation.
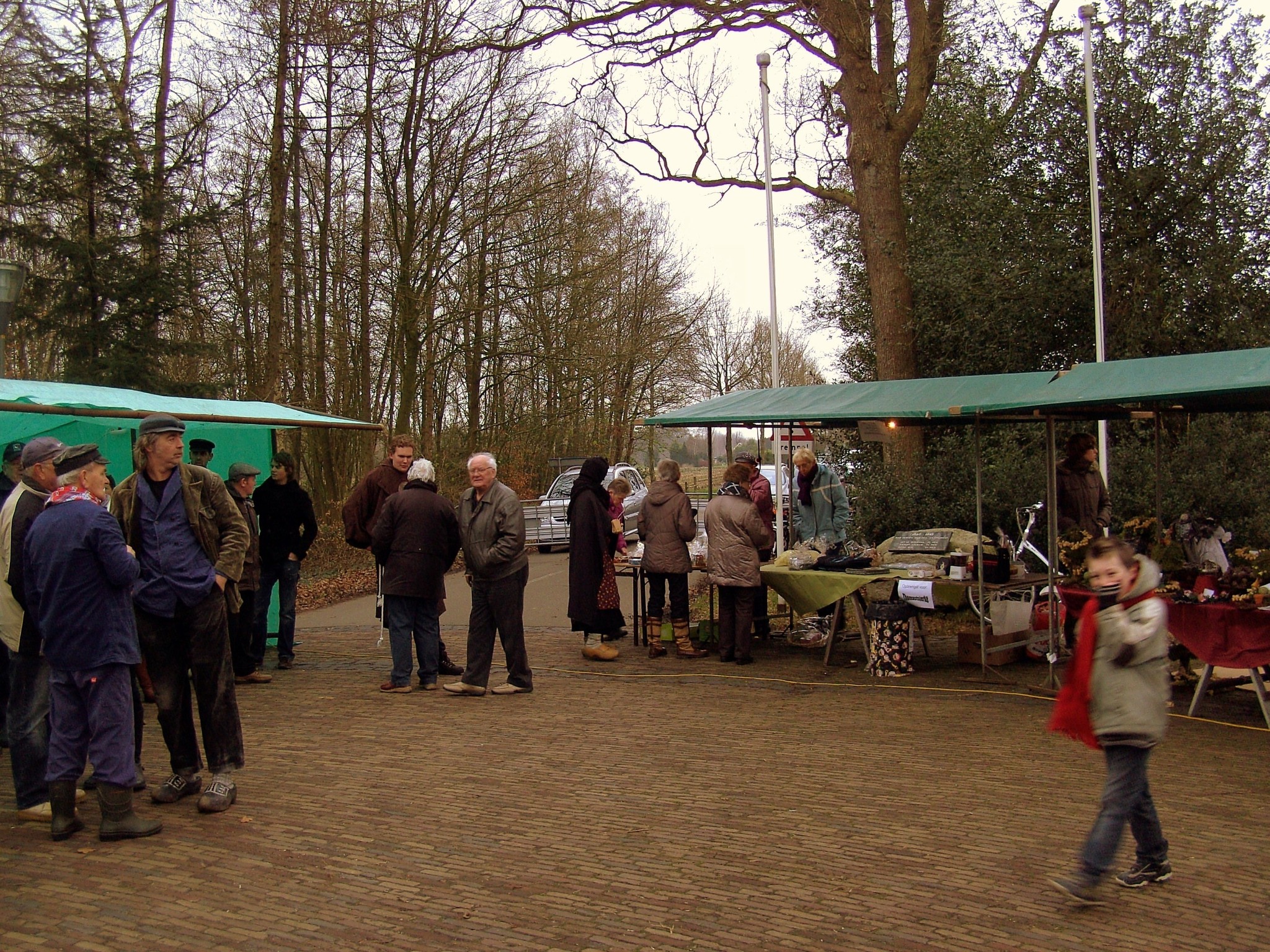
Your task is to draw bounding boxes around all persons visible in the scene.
[0,411,533,840]
[1041,430,1111,645]
[1050,535,1170,903]
[565,448,847,663]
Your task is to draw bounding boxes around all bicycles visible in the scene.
[967,502,1063,626]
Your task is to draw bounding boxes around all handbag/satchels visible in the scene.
[970,541,1010,584]
[596,556,621,609]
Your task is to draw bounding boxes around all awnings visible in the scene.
[0,377,385,430]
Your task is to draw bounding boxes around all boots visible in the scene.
[645,616,667,658]
[671,618,708,658]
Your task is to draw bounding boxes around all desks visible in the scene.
[1048,568,1270,732]
[609,551,1050,677]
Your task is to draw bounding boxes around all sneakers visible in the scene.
[1045,869,1106,906]
[379,679,437,692]
[438,656,464,675]
[1116,853,1173,887]
[443,681,486,696]
[491,683,533,695]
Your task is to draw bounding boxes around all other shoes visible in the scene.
[278,658,292,669]
[759,633,775,648]
[17,763,237,841]
[720,655,757,665]
[607,629,628,641]
[582,644,620,659]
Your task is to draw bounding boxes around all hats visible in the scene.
[189,439,215,453]
[734,451,759,467]
[52,444,112,476]
[21,437,72,468]
[2,442,26,462]
[139,412,186,436]
[228,461,260,482]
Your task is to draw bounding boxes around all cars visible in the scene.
[793,450,862,489]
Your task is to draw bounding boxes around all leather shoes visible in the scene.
[235,670,273,684]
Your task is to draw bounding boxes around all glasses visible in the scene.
[795,462,811,470]
[467,466,493,474]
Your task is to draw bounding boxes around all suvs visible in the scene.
[536,463,648,554]
[758,463,789,499]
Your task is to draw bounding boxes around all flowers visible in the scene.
[1224,542,1269,568]
[1150,574,1270,612]
[1118,514,1181,551]
[1059,549,1091,575]
[1055,525,1095,549]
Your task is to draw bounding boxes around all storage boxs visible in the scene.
[956,630,1020,666]
[640,621,699,642]
[698,618,722,645]
